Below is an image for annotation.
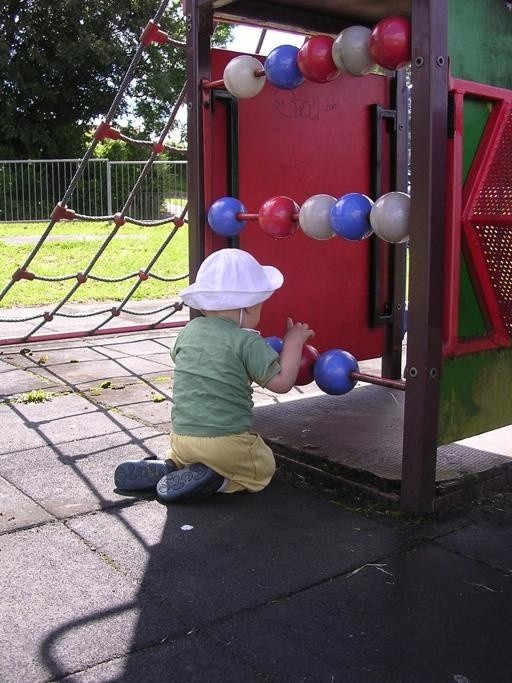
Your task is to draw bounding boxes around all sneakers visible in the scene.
[157,463,225,502]
[113,455,175,491]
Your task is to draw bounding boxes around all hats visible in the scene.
[181,248,284,311]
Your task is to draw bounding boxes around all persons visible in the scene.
[114,248,315,505]
